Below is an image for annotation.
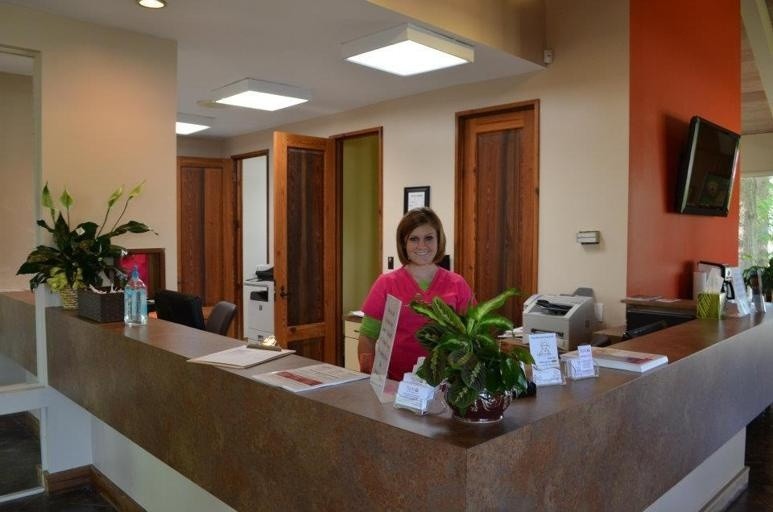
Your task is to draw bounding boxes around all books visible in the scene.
[624,295,662,301]
[560,346,667,373]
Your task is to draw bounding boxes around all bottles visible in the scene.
[123,269,148,326]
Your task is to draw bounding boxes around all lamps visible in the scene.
[340,23,475,77]
[176,113,214,136]
[213,77,309,112]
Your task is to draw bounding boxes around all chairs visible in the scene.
[205,300,239,336]
[153,290,206,329]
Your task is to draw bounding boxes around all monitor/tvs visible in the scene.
[675,116,742,217]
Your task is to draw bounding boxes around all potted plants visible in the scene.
[411,287,536,422]
[16,179,160,308]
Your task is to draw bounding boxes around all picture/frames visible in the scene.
[402,186,430,215]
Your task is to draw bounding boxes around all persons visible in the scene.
[358,206,479,379]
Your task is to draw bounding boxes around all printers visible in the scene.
[522,294,592,351]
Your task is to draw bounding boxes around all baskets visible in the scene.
[78,287,124,322]
